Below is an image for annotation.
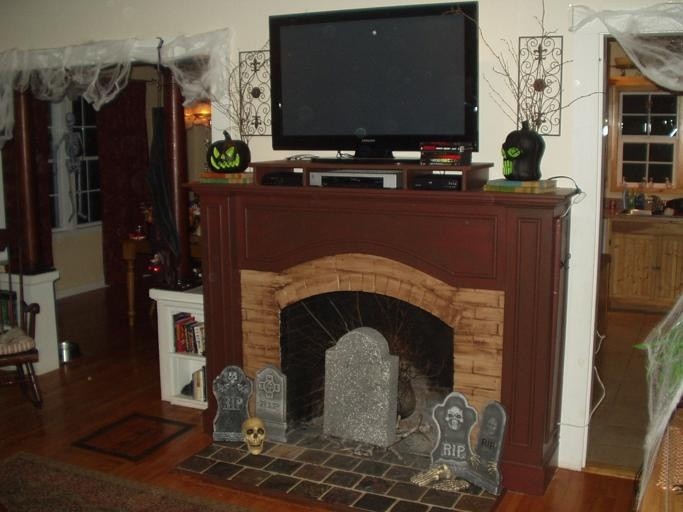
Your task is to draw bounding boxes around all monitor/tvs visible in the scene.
[268,0,480,164]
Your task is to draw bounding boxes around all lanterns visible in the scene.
[205,130,249,173]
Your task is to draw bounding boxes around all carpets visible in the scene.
[0,449,249,512]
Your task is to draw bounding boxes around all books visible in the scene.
[200,172,254,180]
[486,178,557,188]
[172,311,206,404]
[483,185,557,194]
[199,178,254,184]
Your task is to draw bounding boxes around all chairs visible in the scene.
[0,288,44,411]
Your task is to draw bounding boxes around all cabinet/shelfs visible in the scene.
[607,221,683,314]
[147,285,207,412]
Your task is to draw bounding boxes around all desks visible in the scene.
[119,238,204,326]
